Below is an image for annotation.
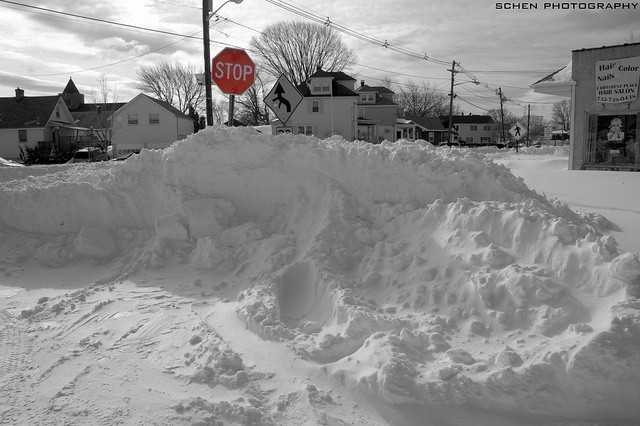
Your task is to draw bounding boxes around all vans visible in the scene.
[73,149,105,163]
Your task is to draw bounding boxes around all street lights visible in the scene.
[203,0,243,125]
[57,127,60,159]
[448,81,480,145]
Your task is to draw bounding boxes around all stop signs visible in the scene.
[212,47,255,95]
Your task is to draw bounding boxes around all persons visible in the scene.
[607,118,625,140]
